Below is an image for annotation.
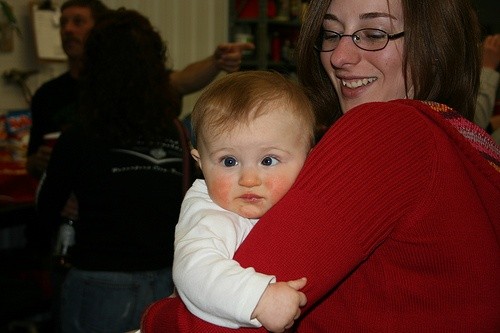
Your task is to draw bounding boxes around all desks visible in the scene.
[0,138,59,204]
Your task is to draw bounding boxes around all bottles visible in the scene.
[51,195,79,270]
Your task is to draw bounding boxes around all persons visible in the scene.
[140,0,500,333]
[26,6,192,333]
[172,69,315,332]
[26,0,258,179]
[473,32,500,131]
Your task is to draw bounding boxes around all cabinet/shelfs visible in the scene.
[229,0,332,95]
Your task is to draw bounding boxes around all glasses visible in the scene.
[312,27,405,52]
